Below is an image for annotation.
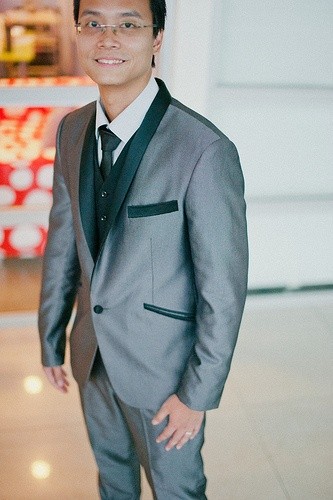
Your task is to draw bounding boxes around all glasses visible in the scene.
[74,16,157,35]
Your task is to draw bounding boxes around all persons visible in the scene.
[37,0,248,500]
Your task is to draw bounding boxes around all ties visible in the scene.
[98,125,122,181]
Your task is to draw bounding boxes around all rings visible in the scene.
[186,432,192,435]
